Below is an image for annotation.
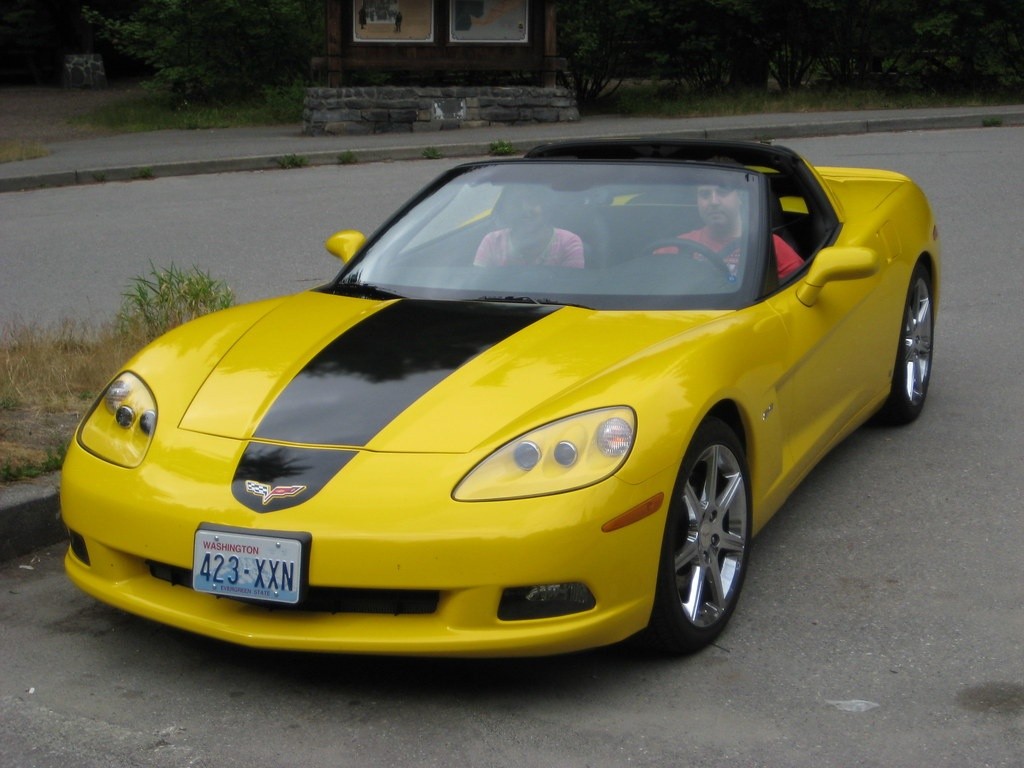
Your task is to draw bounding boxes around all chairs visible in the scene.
[677,177,800,254]
[496,181,608,270]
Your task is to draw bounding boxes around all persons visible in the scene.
[473,185,585,269]
[395,12,402,32]
[653,157,805,284]
[359,6,366,29]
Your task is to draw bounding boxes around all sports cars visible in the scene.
[61,136,940,658]
